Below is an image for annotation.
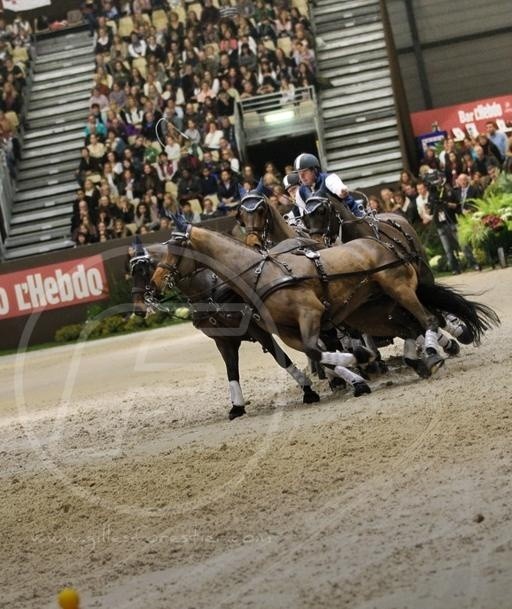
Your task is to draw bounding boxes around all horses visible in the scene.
[125,176,502,421]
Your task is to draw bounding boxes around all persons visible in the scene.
[283,174,301,198]
[294,154,362,238]
[0,3,34,167]
[364,122,512,275]
[284,35,314,97]
[37,3,312,36]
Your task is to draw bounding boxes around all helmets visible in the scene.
[282,173,301,191]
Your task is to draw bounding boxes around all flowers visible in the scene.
[449,174,512,266]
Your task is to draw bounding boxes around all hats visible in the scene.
[294,153,321,169]
